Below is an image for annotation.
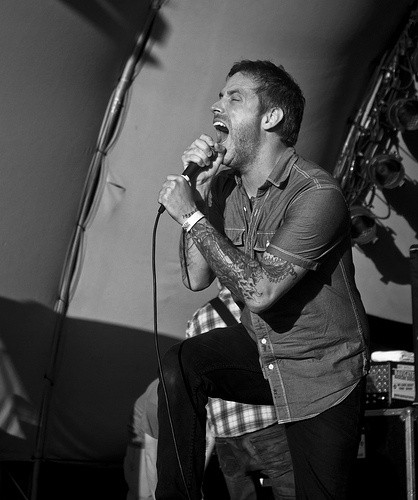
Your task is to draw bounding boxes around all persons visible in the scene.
[183,275,297,500]
[154,58,373,499]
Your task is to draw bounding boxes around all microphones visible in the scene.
[156,143,219,214]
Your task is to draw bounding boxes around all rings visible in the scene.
[181,210,206,234]
